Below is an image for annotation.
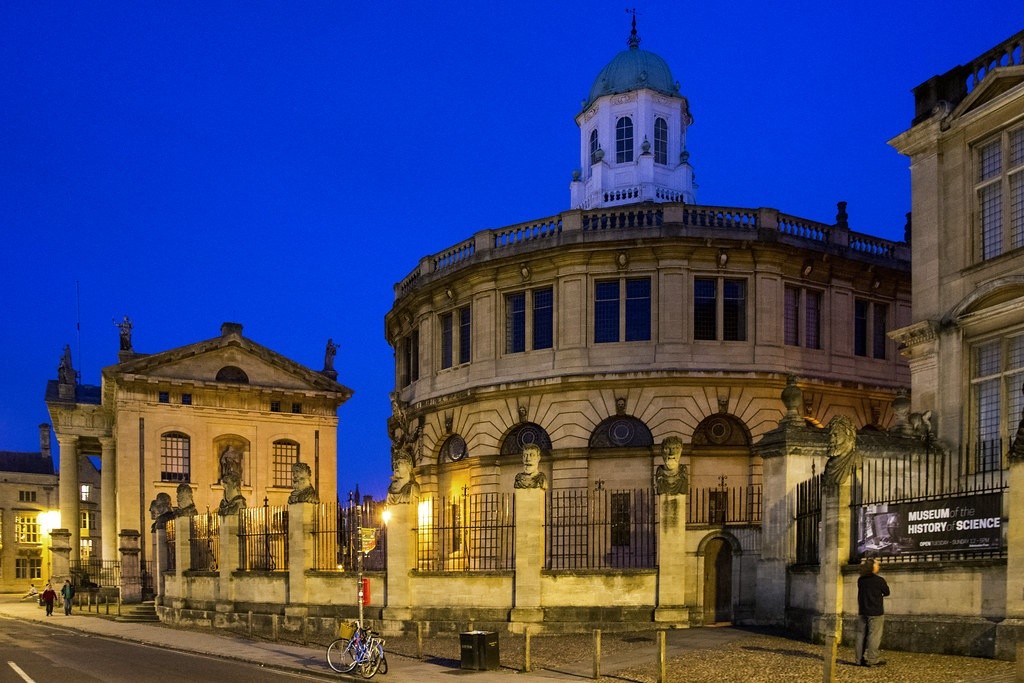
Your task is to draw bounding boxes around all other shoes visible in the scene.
[50,614,53,616]
[69,613,72,615]
[65,613,68,615]
[46,613,49,616]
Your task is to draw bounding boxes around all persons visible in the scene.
[853,558,890,667]
[385,449,421,504]
[43,579,58,616]
[288,462,320,504]
[149,441,248,530]
[514,443,549,490]
[820,414,863,486]
[61,579,75,616]
[23,583,38,599]
[655,435,688,495]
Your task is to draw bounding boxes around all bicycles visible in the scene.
[326,622,385,680]
[359,627,388,674]
